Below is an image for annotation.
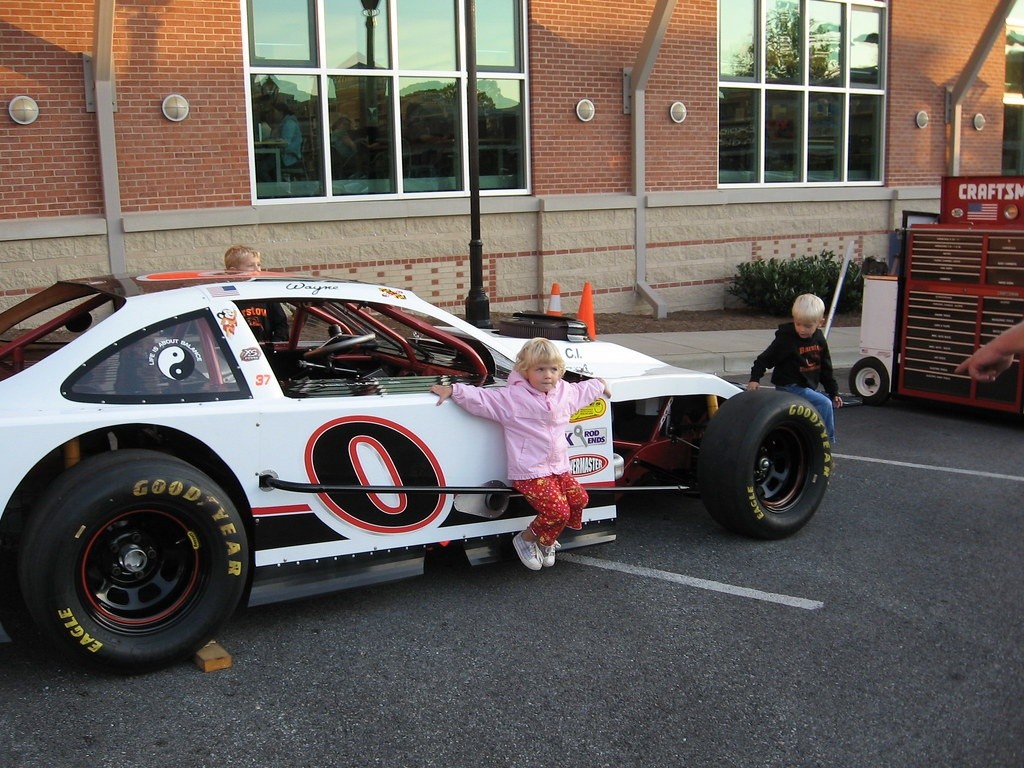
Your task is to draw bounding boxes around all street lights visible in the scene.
[361,0,384,177]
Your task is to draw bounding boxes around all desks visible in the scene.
[253,141,289,182]
[407,139,522,177]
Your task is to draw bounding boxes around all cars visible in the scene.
[0,270,833,674]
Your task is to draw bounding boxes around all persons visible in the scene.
[260,101,301,182]
[430,337,613,571]
[332,118,357,165]
[224,244,289,342]
[954,320,1024,383]
[745,294,843,445]
[354,102,455,177]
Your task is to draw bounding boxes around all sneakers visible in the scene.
[513,530,545,570]
[538,538,562,566]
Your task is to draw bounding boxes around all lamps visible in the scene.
[8,95,39,125]
[161,94,190,122]
[974,113,985,131]
[915,110,929,129]
[669,102,687,124]
[576,98,596,122]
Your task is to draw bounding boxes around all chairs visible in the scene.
[275,139,308,181]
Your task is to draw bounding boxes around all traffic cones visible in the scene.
[545,283,563,317]
[576,281,596,341]
